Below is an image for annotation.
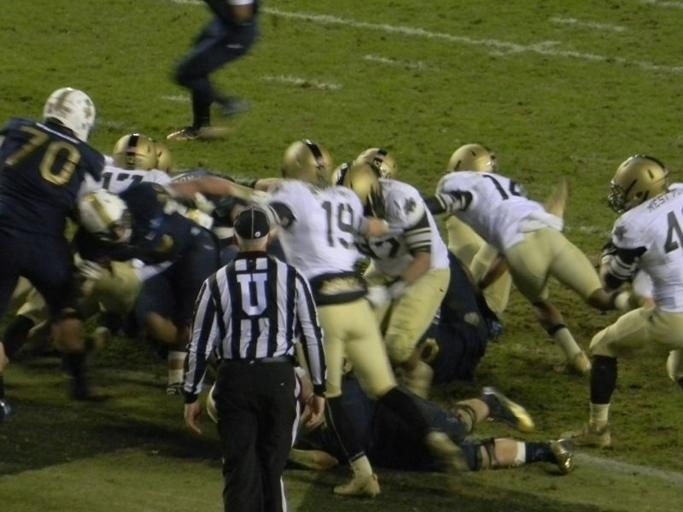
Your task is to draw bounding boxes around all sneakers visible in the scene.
[221,97,250,118]
[68,386,113,401]
[568,350,593,377]
[615,292,641,313]
[481,385,537,432]
[166,126,213,142]
[332,475,381,500]
[548,438,574,473]
[561,427,613,451]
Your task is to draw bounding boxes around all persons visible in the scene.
[166,0,264,145]
[557,152,681,457]
[178,206,329,511]
[2,89,652,502]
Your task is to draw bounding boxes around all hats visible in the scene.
[233,207,271,240]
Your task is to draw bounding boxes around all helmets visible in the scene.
[330,161,383,212]
[280,138,333,187]
[44,86,95,142]
[153,140,174,171]
[355,147,398,179]
[445,142,498,173]
[112,132,158,171]
[606,154,670,214]
